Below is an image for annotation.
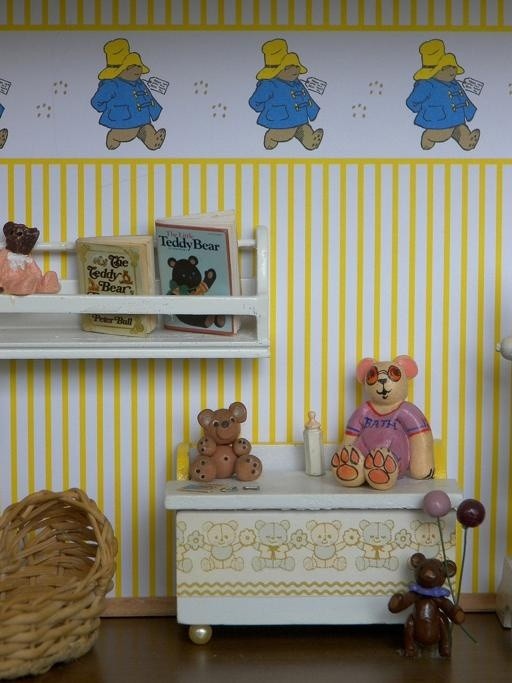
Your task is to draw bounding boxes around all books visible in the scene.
[73,232,159,337]
[152,215,246,336]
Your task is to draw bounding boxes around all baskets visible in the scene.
[0,487,120,681]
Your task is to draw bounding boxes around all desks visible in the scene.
[163,488,460,646]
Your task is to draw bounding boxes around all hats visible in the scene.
[255,38,308,81]
[97,38,151,80]
[412,38,466,81]
